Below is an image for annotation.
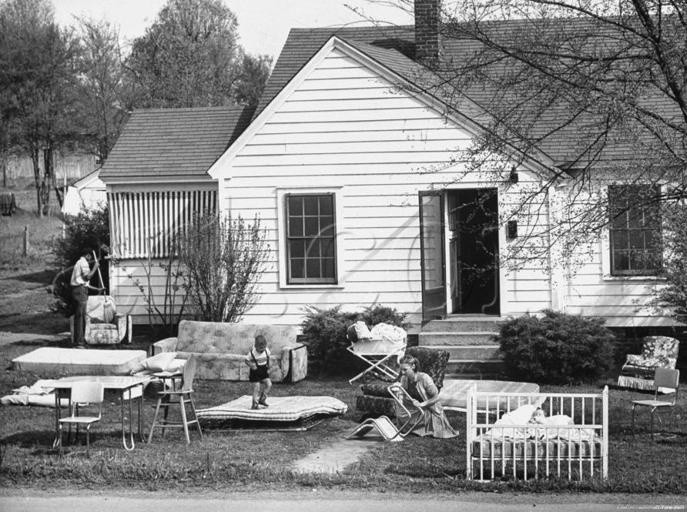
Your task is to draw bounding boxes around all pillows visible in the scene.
[141,352,187,372]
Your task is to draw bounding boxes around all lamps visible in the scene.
[510,165,518,184]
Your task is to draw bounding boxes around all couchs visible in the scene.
[149,318,307,383]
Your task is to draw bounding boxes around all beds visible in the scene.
[462,382,611,482]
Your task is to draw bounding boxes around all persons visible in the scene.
[38,171,50,220]
[394,353,460,439]
[245,334,272,410]
[69,246,106,349]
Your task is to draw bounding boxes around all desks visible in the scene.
[58,380,103,458]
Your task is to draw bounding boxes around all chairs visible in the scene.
[631,368,680,440]
[53,375,148,452]
[618,335,679,390]
[147,352,203,446]
[84,294,126,345]
[346,384,423,442]
[355,346,448,417]
